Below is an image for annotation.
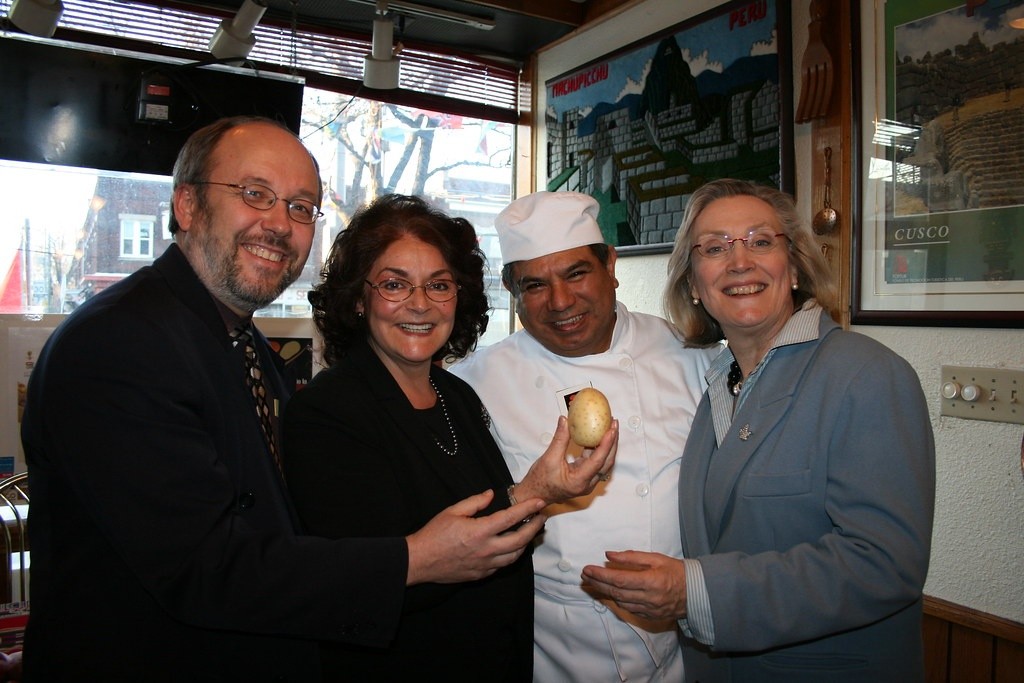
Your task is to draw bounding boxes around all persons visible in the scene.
[20,117,546,683]
[280,196,619,683]
[448,190,725,683]
[581,179,936,683]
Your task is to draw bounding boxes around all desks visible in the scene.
[0,504,30,647]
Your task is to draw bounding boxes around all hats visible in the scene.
[494,191,604,265]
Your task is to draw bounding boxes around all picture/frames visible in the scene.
[847,0,1024,330]
[544,0,796,258]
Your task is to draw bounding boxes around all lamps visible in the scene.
[7,0,64,38]
[363,15,400,90]
[207,0,267,67]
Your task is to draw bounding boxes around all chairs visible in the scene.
[0,472,30,647]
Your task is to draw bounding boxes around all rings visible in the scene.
[597,472,611,482]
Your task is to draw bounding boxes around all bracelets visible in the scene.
[507,485,532,523]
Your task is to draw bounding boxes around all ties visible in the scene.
[230,329,289,483]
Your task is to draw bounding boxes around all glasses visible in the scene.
[190,181,323,224]
[689,229,786,259]
[363,277,462,302]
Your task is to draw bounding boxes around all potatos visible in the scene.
[567,388,612,447]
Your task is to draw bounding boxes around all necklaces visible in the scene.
[727,360,760,396]
[429,375,457,456]
